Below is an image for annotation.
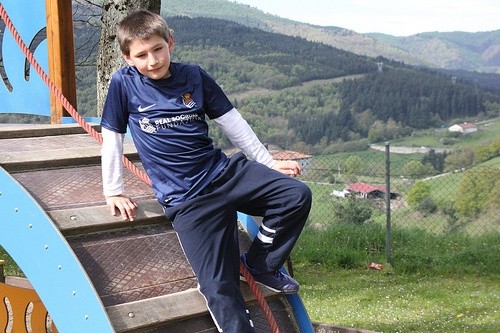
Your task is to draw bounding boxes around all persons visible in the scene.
[97,9,312,333]
